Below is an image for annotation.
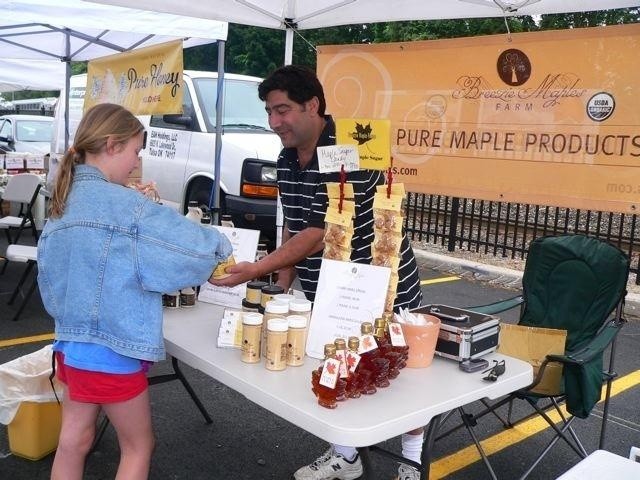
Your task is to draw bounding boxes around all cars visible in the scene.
[0,114,55,158]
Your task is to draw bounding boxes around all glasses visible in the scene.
[481,358,506,383]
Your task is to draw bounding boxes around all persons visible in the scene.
[208,64,425,480]
[36,104,233,480]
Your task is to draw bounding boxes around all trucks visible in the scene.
[50,70,284,246]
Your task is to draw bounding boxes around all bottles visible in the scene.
[349,336,378,395]
[165,291,180,308]
[374,318,401,379]
[335,339,360,398]
[181,286,196,307]
[311,344,347,412]
[290,297,311,326]
[262,301,289,356]
[382,311,409,370]
[360,322,390,388]
[266,318,288,371]
[240,312,263,363]
[287,315,307,366]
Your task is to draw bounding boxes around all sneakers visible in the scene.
[395,462,422,479]
[293,447,365,480]
